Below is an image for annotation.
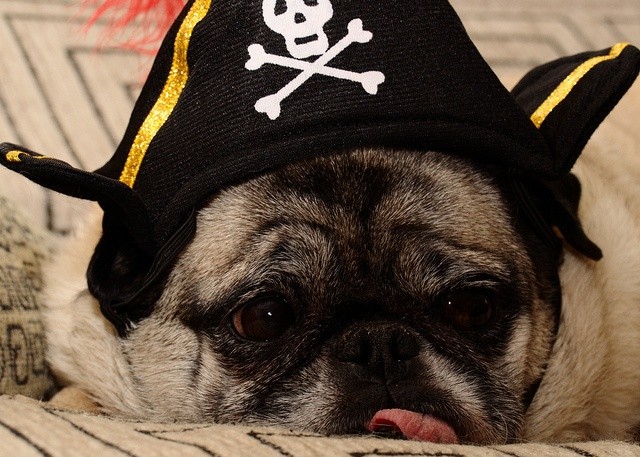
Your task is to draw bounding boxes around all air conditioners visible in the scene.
[0,0,640,445]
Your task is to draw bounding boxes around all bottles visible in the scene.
[0,0,639,339]
